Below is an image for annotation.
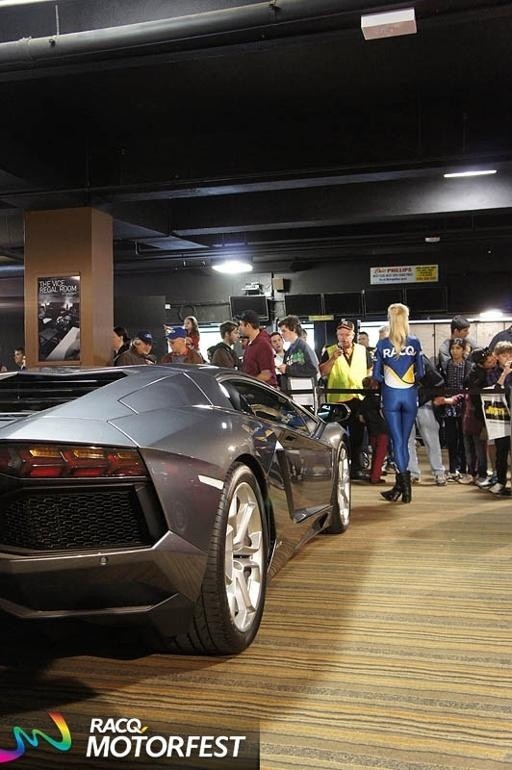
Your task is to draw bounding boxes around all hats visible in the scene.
[136,330,158,344]
[236,310,259,323]
[336,320,354,330]
[163,326,187,339]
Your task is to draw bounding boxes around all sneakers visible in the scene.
[413,471,512,498]
[372,479,385,484]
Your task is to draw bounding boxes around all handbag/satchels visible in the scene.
[479,383,511,441]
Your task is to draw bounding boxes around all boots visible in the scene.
[398,471,411,504]
[381,473,402,503]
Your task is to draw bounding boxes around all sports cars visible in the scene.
[1,363,356,660]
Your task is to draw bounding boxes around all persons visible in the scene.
[21,354,26,370]
[14,346,25,371]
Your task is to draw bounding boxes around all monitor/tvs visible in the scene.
[229,296,270,323]
